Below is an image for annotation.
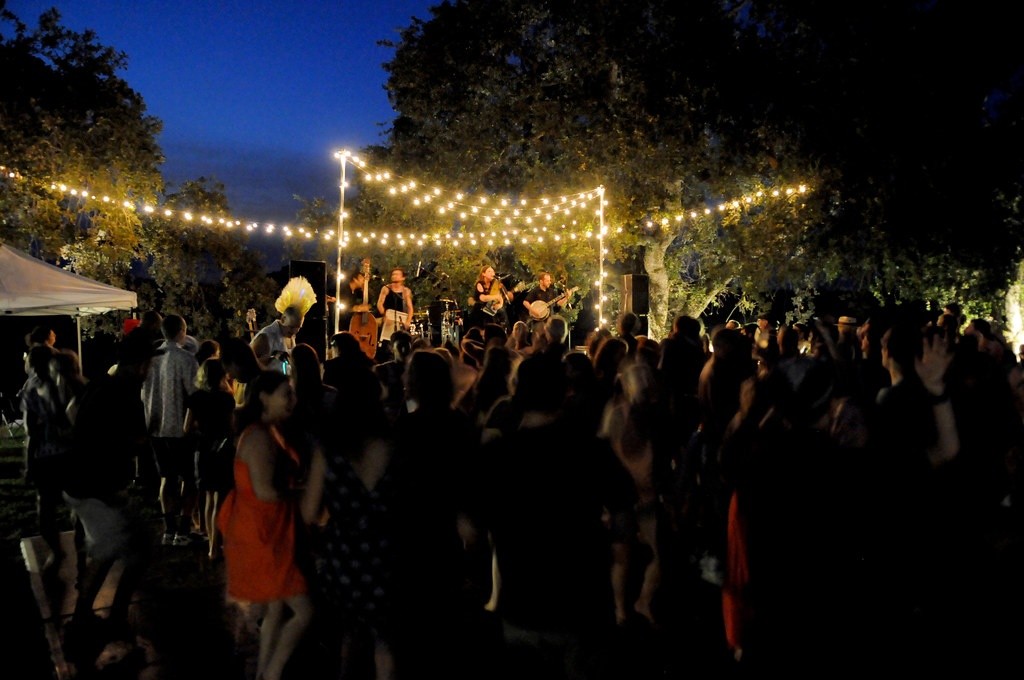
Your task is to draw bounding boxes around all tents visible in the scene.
[0,242,140,378]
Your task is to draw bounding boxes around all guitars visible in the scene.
[529,286,580,321]
[245,307,258,340]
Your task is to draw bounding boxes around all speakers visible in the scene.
[290,260,328,364]
[619,274,650,337]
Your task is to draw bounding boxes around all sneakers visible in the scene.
[161,533,193,546]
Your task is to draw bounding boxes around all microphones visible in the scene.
[557,281,565,287]
[417,261,421,277]
[493,275,500,279]
[442,272,449,278]
[372,275,385,281]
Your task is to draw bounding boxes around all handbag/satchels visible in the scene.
[699,555,724,586]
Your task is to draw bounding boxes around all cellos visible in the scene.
[348,259,378,359]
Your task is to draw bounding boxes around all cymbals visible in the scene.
[439,299,454,303]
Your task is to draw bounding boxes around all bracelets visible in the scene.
[924,384,950,405]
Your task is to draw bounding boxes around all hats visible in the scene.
[832,316,859,327]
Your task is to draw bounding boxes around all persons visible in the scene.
[377,267,414,342]
[18,277,1023,680]
[219,370,312,679]
[474,265,512,335]
[140,314,200,545]
[457,352,640,679]
[523,273,572,324]
[329,272,372,347]
[303,368,414,680]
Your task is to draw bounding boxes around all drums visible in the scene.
[410,312,429,331]
[450,308,469,325]
